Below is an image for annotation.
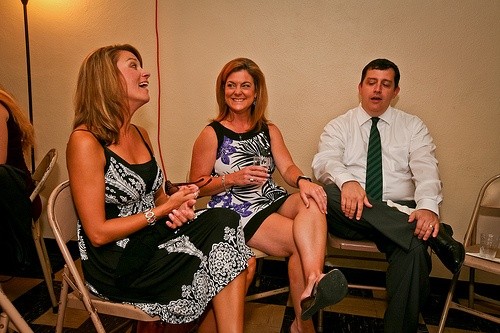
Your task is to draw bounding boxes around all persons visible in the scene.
[66,43,257,333]
[0,86,43,276]
[311,58,465,333]
[189,56,349,333]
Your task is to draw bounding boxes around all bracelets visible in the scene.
[221,174,229,189]
[142,208,157,226]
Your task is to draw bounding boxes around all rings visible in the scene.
[250,176,254,182]
[429,224,434,229]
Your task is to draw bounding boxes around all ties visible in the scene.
[365,117,383,202]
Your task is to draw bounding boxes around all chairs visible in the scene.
[438,174,500,333]
[186,168,290,301]
[29,148,59,313]
[313,176,432,333]
[47,179,160,333]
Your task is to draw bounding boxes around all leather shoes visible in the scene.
[432,223,465,274]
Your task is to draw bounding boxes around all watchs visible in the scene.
[296,175,312,188]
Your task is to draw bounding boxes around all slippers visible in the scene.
[300,269,348,321]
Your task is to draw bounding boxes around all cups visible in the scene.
[479,233,500,259]
[253,156,270,184]
